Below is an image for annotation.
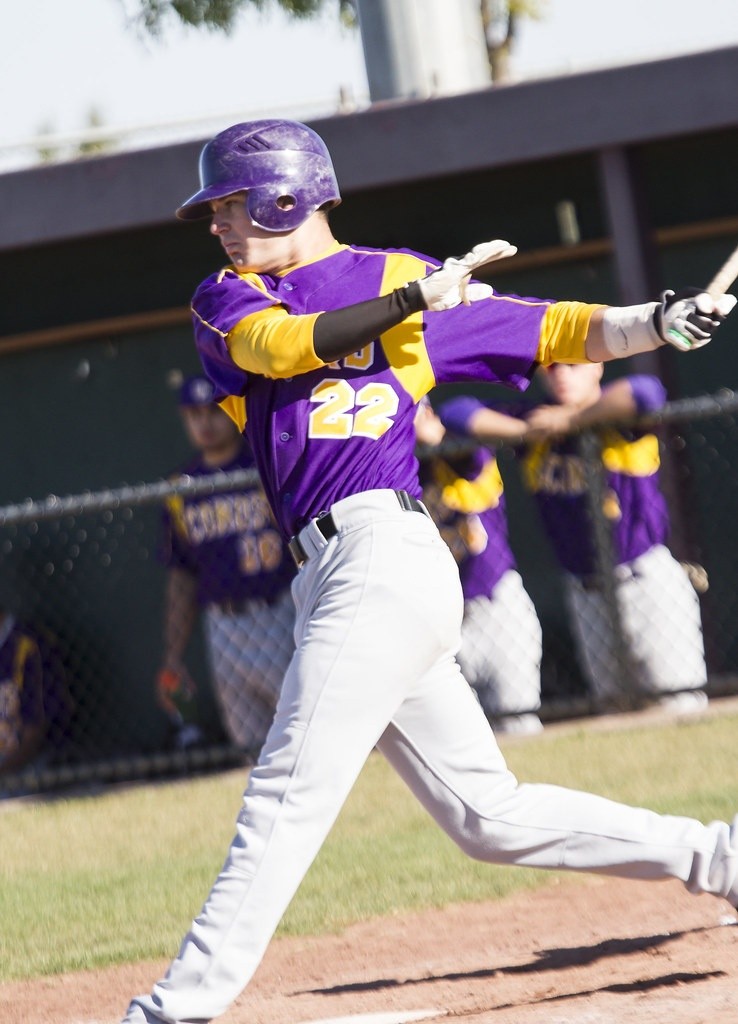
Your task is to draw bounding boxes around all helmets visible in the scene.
[173,117,341,233]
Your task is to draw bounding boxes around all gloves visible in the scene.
[681,562,709,592]
[655,289,737,352]
[157,667,194,718]
[418,237,516,312]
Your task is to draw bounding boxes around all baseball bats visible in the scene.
[664,245,738,352]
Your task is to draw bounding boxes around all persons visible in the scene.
[120,121,738,1024]
[0,601,44,800]
[156,349,711,768]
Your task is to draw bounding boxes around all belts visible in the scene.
[289,490,424,564]
[580,561,637,592]
[219,594,279,615]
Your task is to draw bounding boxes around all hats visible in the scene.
[173,374,226,405]
